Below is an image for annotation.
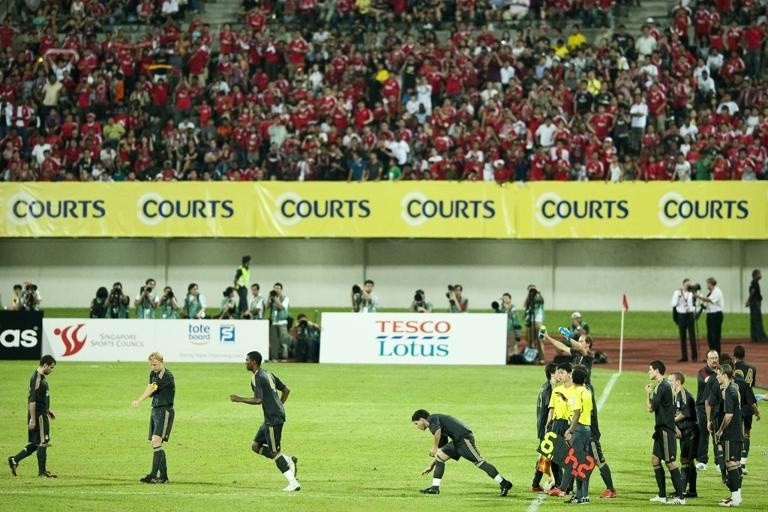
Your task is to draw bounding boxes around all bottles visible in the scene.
[558,327,573,339]
[537,325,546,340]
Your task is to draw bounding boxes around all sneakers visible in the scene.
[420,487,439,494]
[283,483,299,491]
[499,456,748,507]
[141,475,157,482]
[8,457,18,477]
[289,457,298,477]
[152,478,169,484]
[38,471,57,478]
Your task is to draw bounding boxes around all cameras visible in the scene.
[446,285,455,305]
[167,291,175,299]
[688,283,702,294]
[352,284,360,295]
[269,290,276,305]
[490,296,504,309]
[526,289,538,302]
[299,319,308,328]
[139,286,153,295]
[414,293,423,308]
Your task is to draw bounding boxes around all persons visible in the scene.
[290,314,320,362]
[522,284,546,363]
[90,286,107,316]
[530,313,618,503]
[229,352,299,491]
[180,284,207,319]
[645,347,758,506]
[695,277,725,362]
[412,407,512,497]
[244,283,266,318]
[231,255,250,318]
[0,0,767,181]
[103,282,131,318]
[219,288,237,320]
[19,280,42,310]
[410,286,434,314]
[494,291,519,361]
[745,270,767,342]
[6,355,58,479]
[353,279,377,311]
[135,278,155,319]
[134,351,175,482]
[157,286,180,319]
[672,279,696,361]
[268,283,290,361]
[11,282,20,309]
[447,285,469,313]
